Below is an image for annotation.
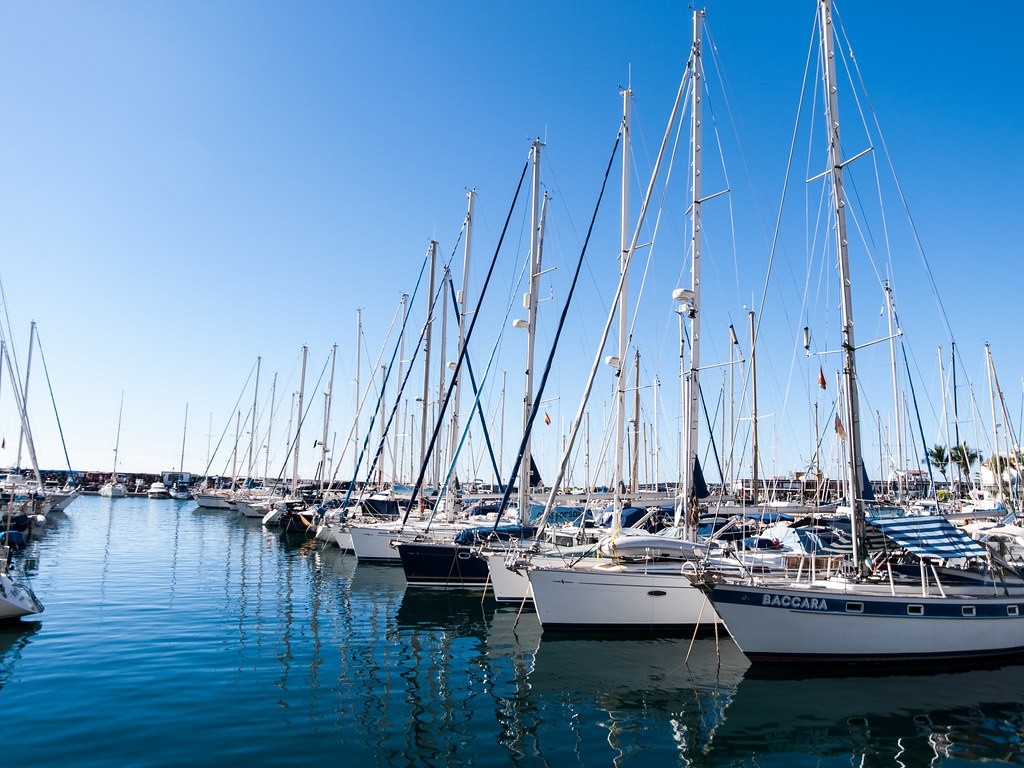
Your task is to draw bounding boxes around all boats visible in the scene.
[147,481,168,498]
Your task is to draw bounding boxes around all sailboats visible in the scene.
[510,642,751,768]
[528,3,758,642]
[188,344,359,553]
[171,401,191,502]
[95,388,129,498]
[389,185,497,590]
[347,292,417,567]
[483,136,585,605]
[705,675,1024,768]
[686,0,1024,680]
[0,276,83,621]
[394,590,499,695]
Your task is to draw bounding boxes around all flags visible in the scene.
[834,412,847,441]
[818,365,826,391]
[544,412,551,426]
